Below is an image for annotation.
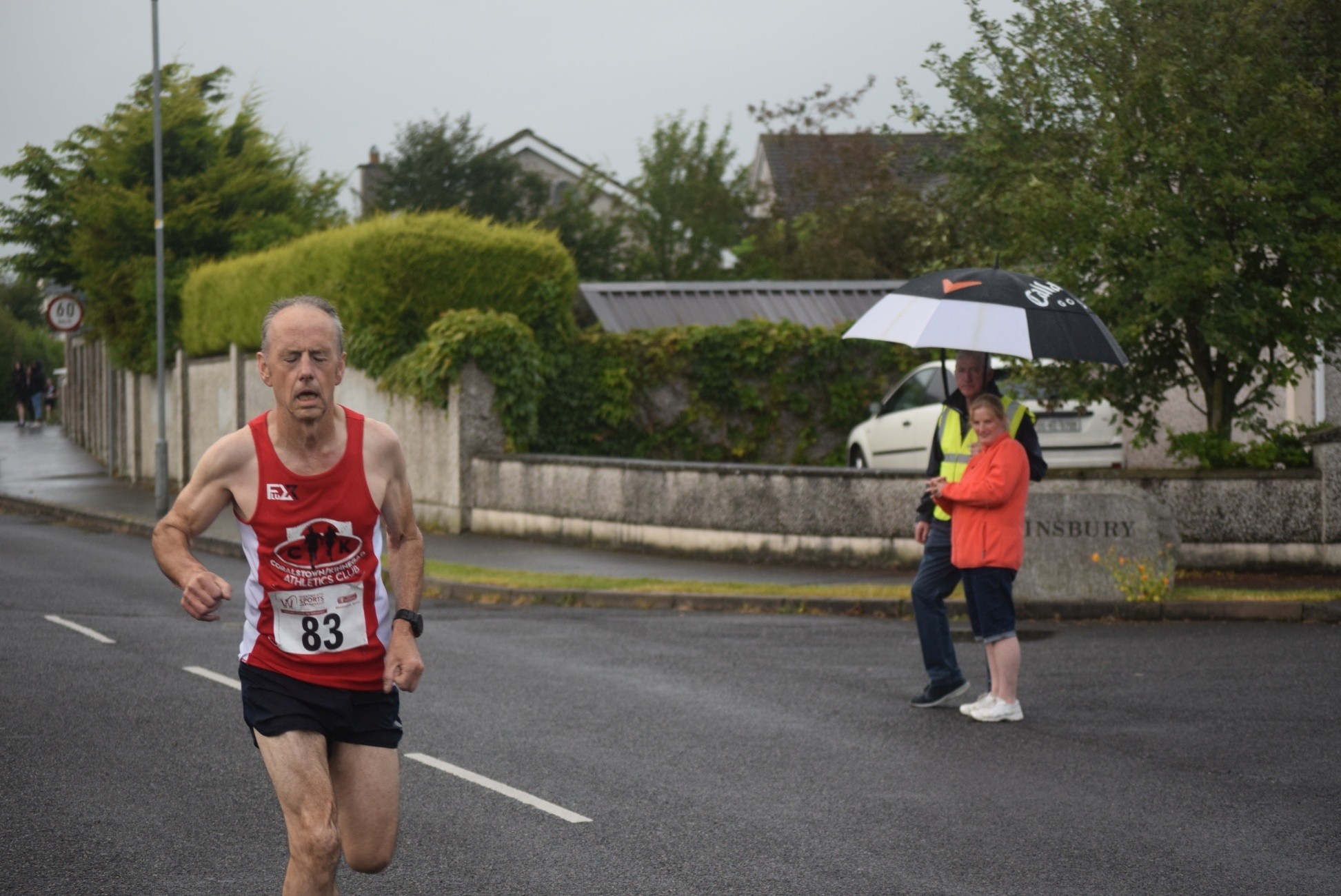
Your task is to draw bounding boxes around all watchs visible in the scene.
[391,609,423,638]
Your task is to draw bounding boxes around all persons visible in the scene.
[9,361,58,432]
[148,293,425,896]
[925,393,1029,723]
[908,346,1048,707]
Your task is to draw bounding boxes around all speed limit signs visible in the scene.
[48,295,86,332]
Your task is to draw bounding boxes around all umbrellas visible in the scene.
[841,253,1130,392]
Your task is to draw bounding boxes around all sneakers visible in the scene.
[960,692,996,717]
[909,677,970,708]
[970,697,1024,723]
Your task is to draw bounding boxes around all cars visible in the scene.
[846,353,1127,469]
